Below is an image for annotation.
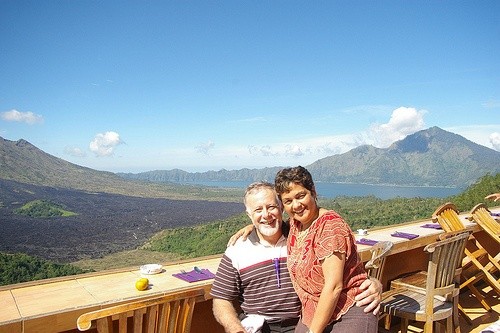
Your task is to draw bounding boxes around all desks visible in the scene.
[0,205,500,333]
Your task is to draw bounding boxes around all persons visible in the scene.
[227,166,379,333]
[485,193,500,202]
[209,182,382,333]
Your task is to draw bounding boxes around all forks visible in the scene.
[181,270,199,281]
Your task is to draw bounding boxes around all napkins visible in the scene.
[491,213,500,216]
[391,232,419,240]
[355,238,378,246]
[420,224,442,229]
[172,269,216,283]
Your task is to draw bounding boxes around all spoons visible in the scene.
[194,267,212,278]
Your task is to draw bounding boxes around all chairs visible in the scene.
[390,230,473,333]
[76,288,207,333]
[431,201,500,325]
[468,203,500,299]
[365,241,393,282]
[380,232,469,333]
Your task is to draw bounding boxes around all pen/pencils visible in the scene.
[274,257,280,286]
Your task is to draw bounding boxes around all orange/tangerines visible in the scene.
[135,278,149,291]
